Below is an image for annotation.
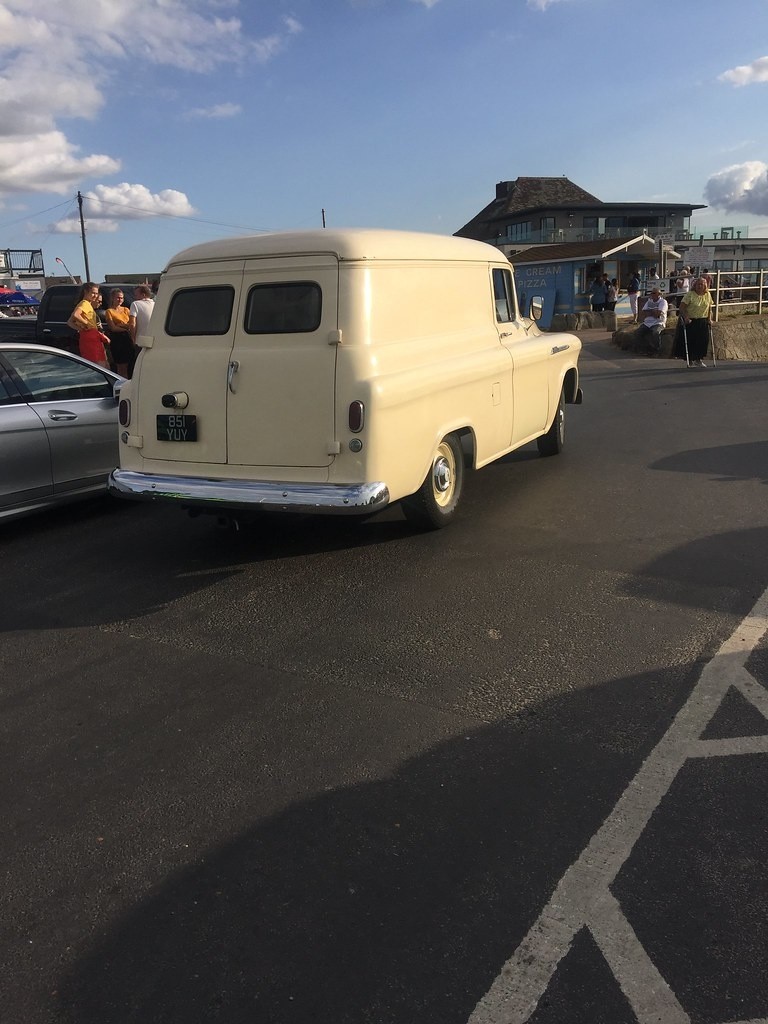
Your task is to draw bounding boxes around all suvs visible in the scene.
[110,228,585,529]
[33,282,158,357]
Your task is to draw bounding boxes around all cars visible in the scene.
[1,344,138,520]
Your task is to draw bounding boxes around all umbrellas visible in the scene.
[0,288,41,307]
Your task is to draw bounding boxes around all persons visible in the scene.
[106,288,135,378]
[129,285,155,359]
[670,265,713,305]
[638,288,668,358]
[67,281,111,370]
[590,273,618,311]
[627,271,642,324]
[92,293,111,345]
[0,306,38,318]
[673,277,714,367]
[650,267,659,279]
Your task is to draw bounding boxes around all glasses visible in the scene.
[651,292,656,294]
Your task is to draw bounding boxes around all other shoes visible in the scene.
[694,360,707,368]
[637,351,648,356]
